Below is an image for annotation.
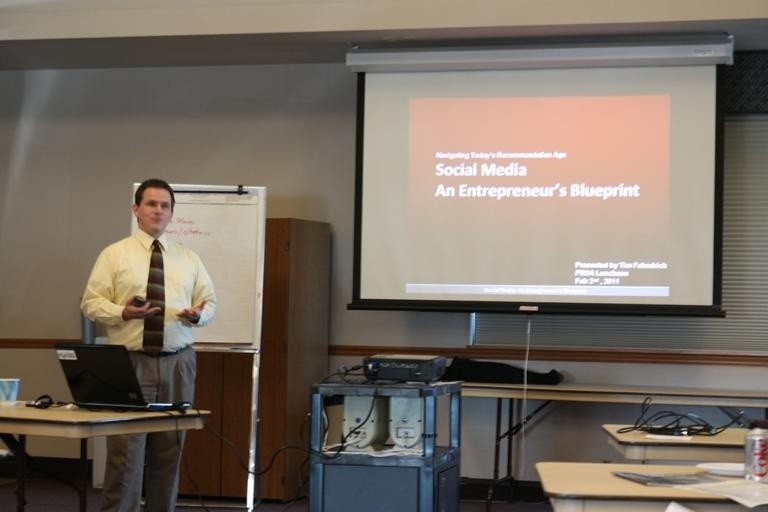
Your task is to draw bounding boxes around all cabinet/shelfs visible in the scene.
[308,380,462,512]
[179,215,331,499]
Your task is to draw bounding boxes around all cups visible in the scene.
[0,379,21,402]
[81,314,95,344]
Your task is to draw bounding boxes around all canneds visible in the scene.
[745,430,768,483]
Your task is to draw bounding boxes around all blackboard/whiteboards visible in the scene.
[130,182,267,353]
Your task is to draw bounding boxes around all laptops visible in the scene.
[53,342,176,412]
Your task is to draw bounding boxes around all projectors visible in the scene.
[362,354,446,383]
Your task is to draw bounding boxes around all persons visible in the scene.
[80,179,217,512]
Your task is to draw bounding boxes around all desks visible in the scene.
[602,423,749,463]
[535,461,768,512]
[461,381,768,512]
[0,399,212,512]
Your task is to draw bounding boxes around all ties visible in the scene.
[143,240,165,357]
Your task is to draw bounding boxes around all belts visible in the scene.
[137,346,189,356]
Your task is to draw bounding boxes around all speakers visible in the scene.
[387,398,424,453]
[338,395,389,451]
